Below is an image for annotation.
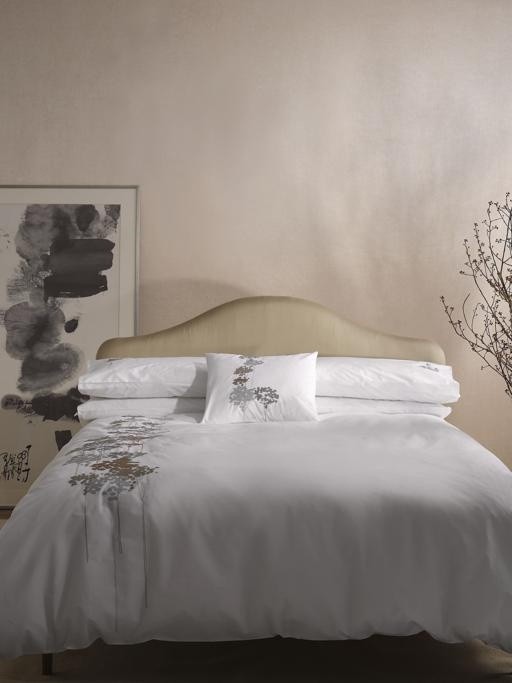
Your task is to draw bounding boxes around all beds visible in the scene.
[1,296,511,675]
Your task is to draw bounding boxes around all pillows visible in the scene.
[199,352,319,421]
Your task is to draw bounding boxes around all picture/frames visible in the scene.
[0,184,139,508]
